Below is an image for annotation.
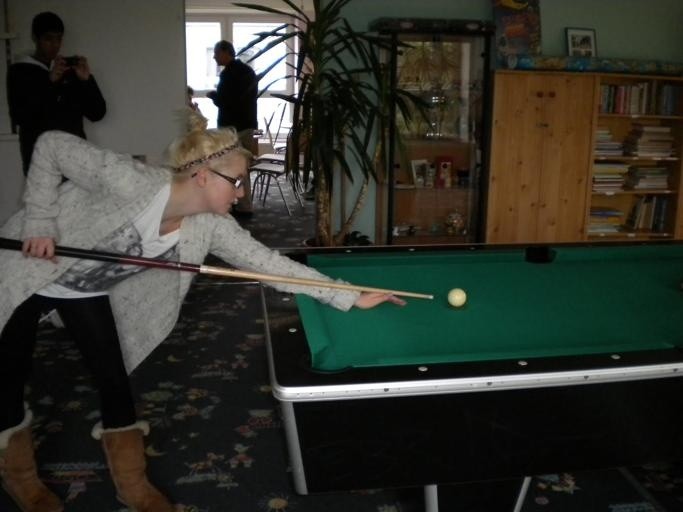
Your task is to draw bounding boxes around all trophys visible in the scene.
[421,95,447,139]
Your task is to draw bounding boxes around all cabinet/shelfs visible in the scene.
[587,73,683,241]
[371,15,489,245]
[480,69,599,244]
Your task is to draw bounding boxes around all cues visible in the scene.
[0,239,434,300]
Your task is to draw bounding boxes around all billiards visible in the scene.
[448,288,467,306]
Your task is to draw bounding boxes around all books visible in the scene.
[593,124,625,157]
[624,194,668,231]
[591,159,632,194]
[624,164,672,190]
[657,84,682,116]
[587,206,625,234]
[598,81,652,116]
[621,122,678,158]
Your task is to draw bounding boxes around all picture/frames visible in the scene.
[566,26,598,59]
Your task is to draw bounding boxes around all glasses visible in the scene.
[191,164,246,189]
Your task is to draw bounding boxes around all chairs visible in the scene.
[257,124,304,200]
[247,133,303,216]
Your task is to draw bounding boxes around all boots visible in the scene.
[0,405,65,511]
[90,422,175,512]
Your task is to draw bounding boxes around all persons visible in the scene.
[1,101,407,511]
[204,40,258,219]
[5,11,106,185]
[187,86,201,114]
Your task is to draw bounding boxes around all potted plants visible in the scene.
[233,1,436,250]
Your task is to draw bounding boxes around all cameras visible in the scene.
[55,56,81,67]
